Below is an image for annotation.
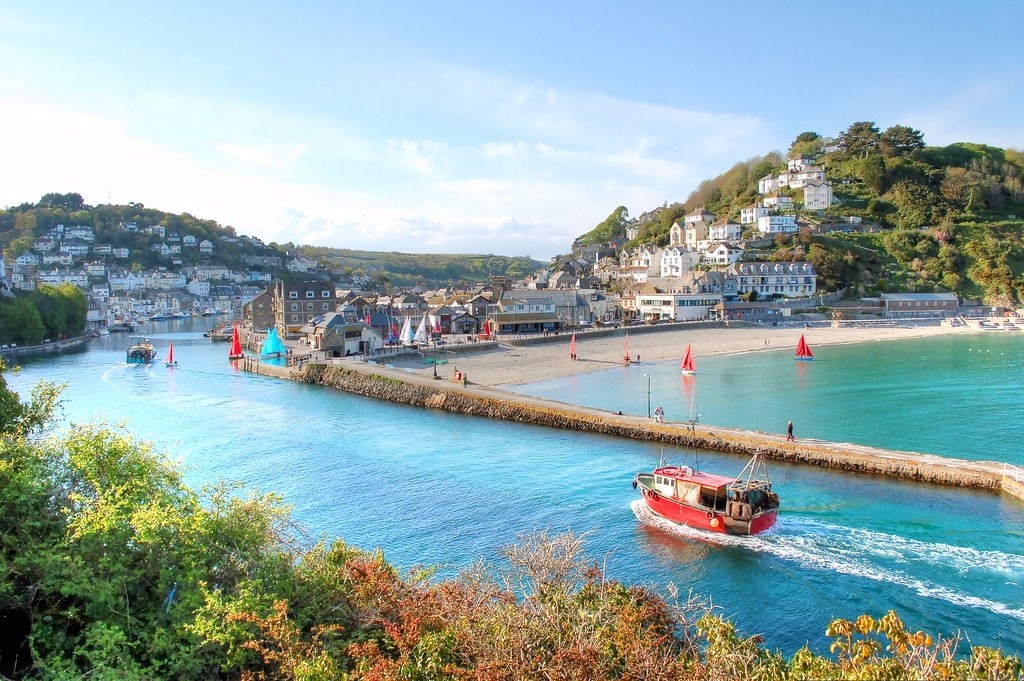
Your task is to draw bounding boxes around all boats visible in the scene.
[150,299,246,336]
[89,317,148,338]
[631,414,780,536]
[124,334,158,365]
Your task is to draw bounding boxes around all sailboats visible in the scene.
[622,331,631,364]
[680,343,696,375]
[794,333,814,362]
[569,331,579,363]
[164,340,179,367]
[227,325,245,360]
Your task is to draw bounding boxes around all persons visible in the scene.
[787,420,796,442]
[276,338,318,370]
[654,406,664,423]
[636,354,640,364]
[461,372,468,389]
[764,338,770,346]
